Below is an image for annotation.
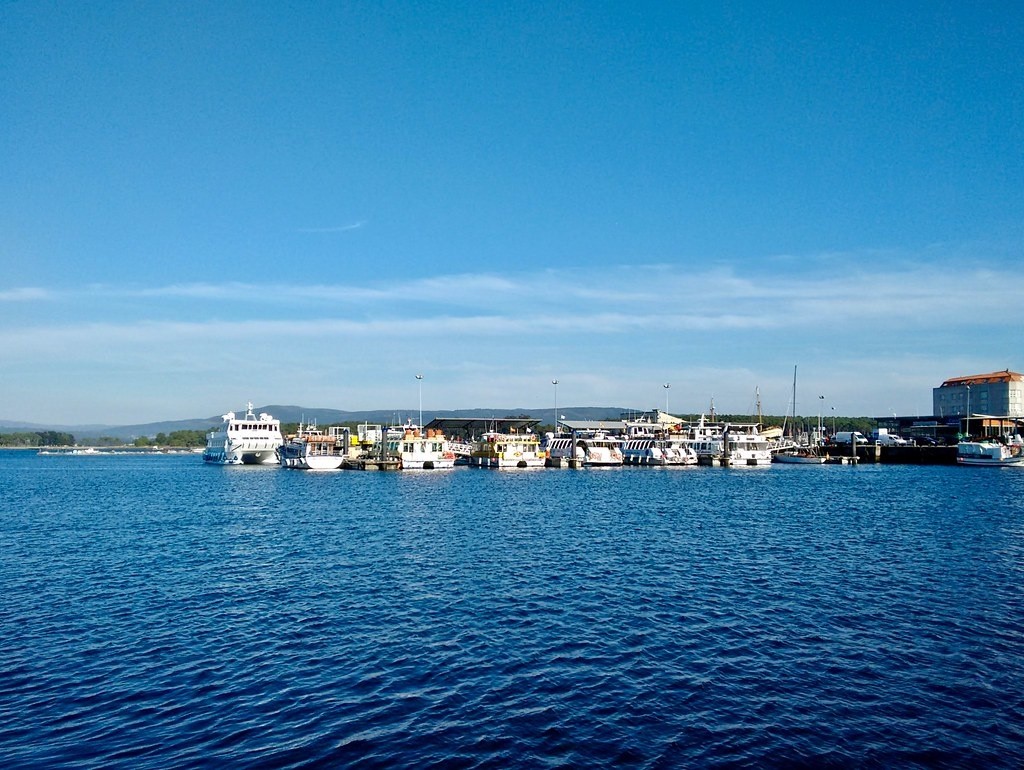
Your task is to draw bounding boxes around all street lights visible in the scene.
[965,385,970,437]
[664,382,671,415]
[552,379,560,432]
[415,375,424,434]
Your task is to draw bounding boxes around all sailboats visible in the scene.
[774,365,827,463]
[745,385,783,438]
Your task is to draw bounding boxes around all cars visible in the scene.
[913,436,939,447]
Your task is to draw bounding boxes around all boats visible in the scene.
[548,425,625,467]
[200,401,284,465]
[679,412,773,466]
[469,413,547,468]
[376,415,455,468]
[956,434,1024,467]
[342,427,402,471]
[621,417,699,465]
[274,422,343,470]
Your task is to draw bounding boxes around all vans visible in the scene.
[836,432,868,445]
[878,434,907,447]
